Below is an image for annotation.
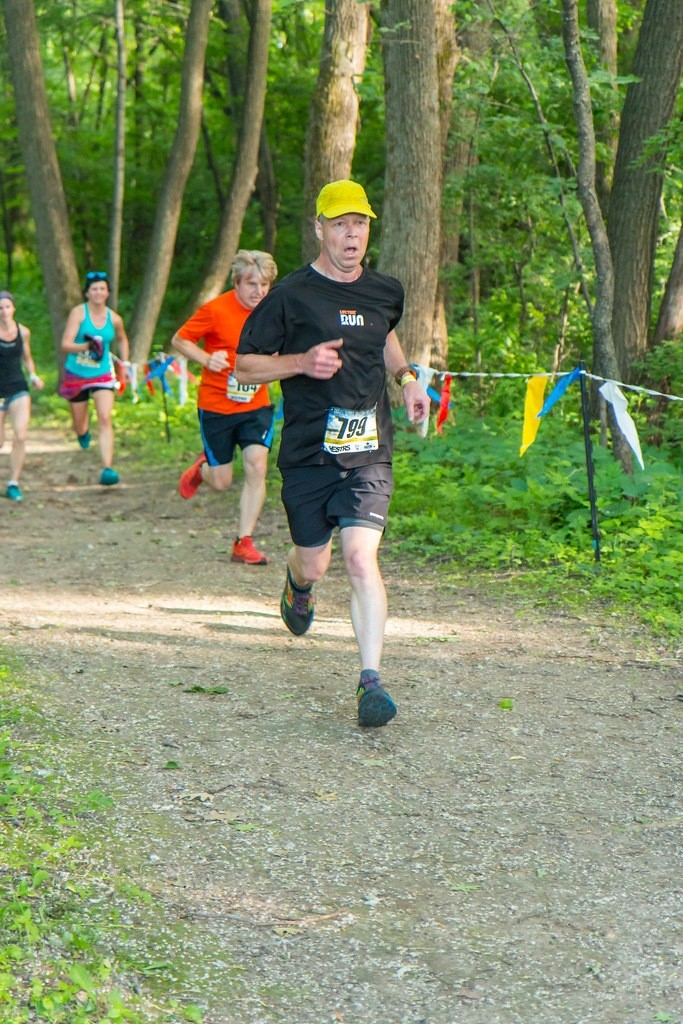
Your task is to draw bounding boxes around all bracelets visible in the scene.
[29,373,38,380]
[395,365,418,389]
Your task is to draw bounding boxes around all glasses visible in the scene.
[85,272,107,279]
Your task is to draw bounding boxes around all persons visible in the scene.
[234,180,431,728]
[61,272,132,485]
[170,249,278,565]
[0,290,45,500]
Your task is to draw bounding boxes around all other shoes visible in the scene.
[98,467,119,486]
[6,485,23,501]
[78,428,92,449]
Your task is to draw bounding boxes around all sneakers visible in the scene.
[356,677,397,727]
[280,563,314,636]
[231,537,269,565]
[179,453,209,500]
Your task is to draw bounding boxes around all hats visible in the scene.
[316,179,378,220]
